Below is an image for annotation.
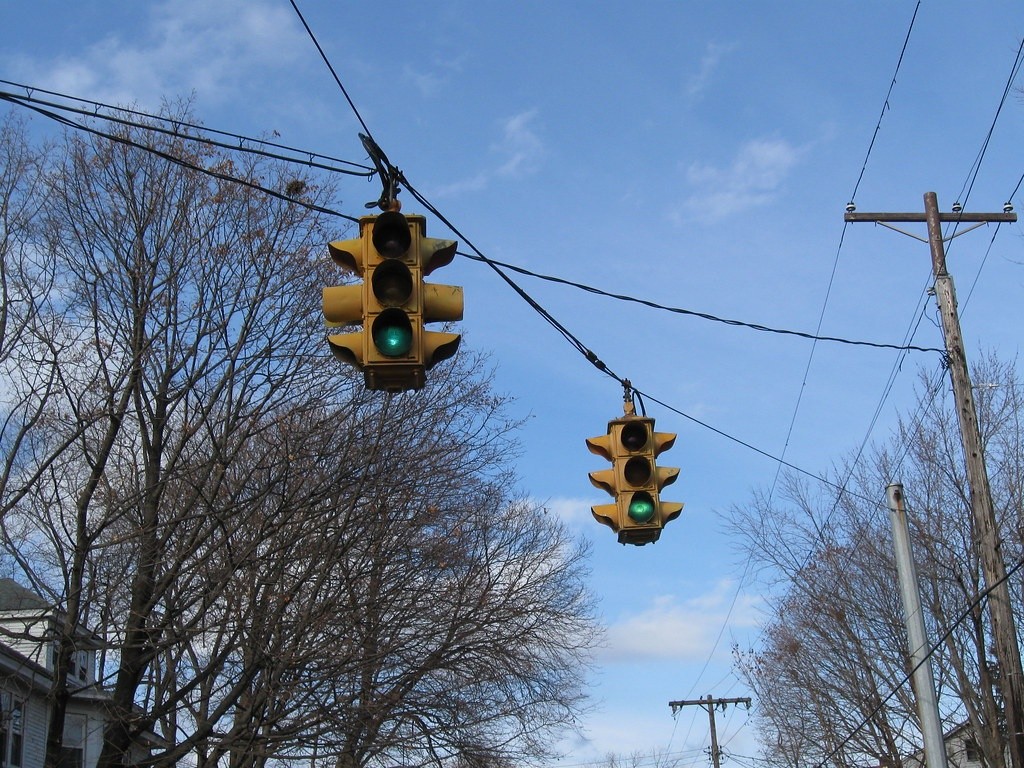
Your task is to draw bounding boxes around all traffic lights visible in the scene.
[584,423,622,535]
[615,416,664,534]
[321,224,367,370]
[417,214,465,375]
[359,211,419,364]
[646,417,684,537]
